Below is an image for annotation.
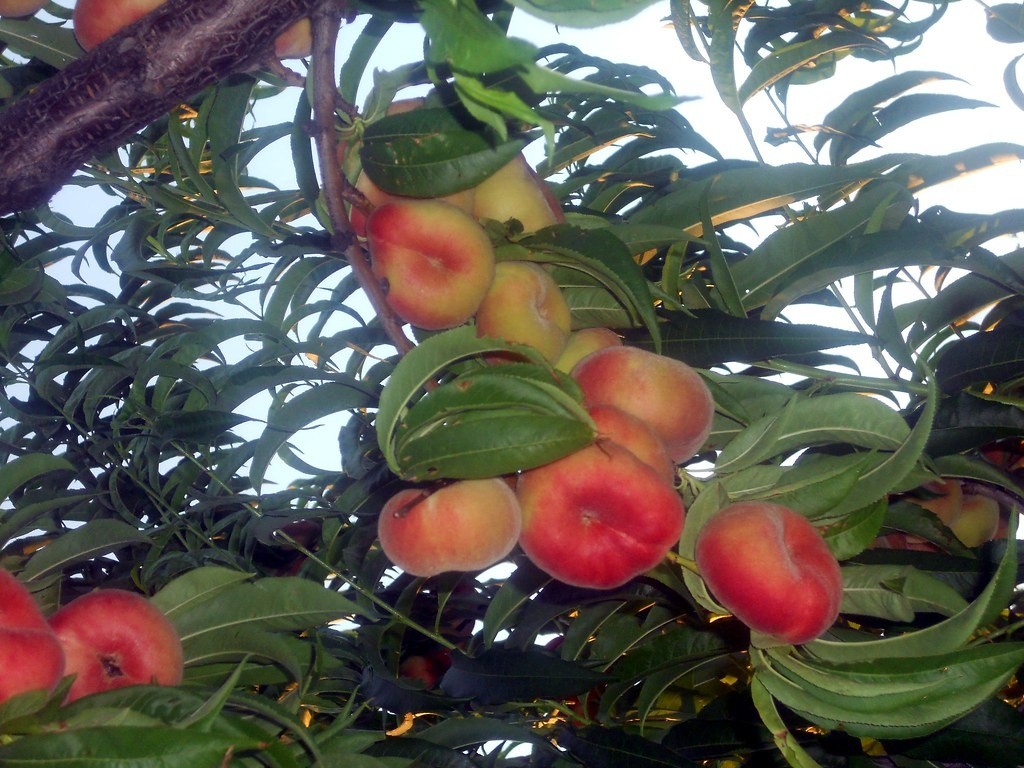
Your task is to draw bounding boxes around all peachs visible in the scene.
[327,98,1024,642]
[0,0,314,59]
[0,564,183,706]
